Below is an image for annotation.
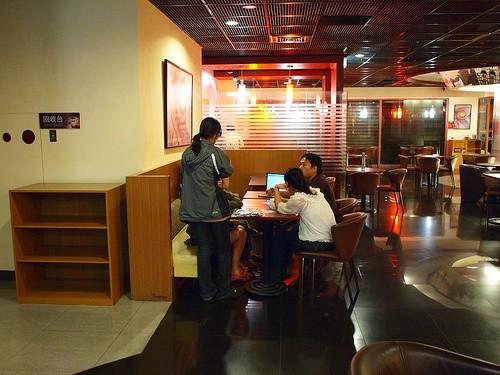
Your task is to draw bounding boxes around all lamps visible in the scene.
[363,105,368,119]
[238,70,247,103]
[285,65,294,105]
[430,104,435,119]
[397,106,402,119]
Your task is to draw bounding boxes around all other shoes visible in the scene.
[200,227,264,303]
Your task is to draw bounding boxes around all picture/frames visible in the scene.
[164,58,192,149]
[453,104,472,130]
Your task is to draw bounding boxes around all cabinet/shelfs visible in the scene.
[448,138,482,175]
[8,182,127,306]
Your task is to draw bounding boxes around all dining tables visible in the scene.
[231,189,301,298]
[408,154,445,186]
[478,162,500,171]
[247,173,267,191]
[346,165,386,208]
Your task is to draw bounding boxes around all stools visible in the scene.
[481,189,500,232]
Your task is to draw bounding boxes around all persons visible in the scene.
[224,124,244,151]
[185,177,252,284]
[178,117,238,301]
[265,153,338,219]
[272,166,338,280]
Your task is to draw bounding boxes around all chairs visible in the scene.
[415,146,434,155]
[346,171,379,214]
[417,157,441,189]
[460,164,489,203]
[398,154,420,185]
[295,211,367,304]
[376,168,409,214]
[335,197,359,215]
[440,155,457,189]
[400,146,410,155]
[351,340,500,375]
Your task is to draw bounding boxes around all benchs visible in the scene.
[169,196,198,278]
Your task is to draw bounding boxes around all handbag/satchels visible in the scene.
[215,186,232,217]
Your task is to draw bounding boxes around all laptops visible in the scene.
[258,173,290,198]
